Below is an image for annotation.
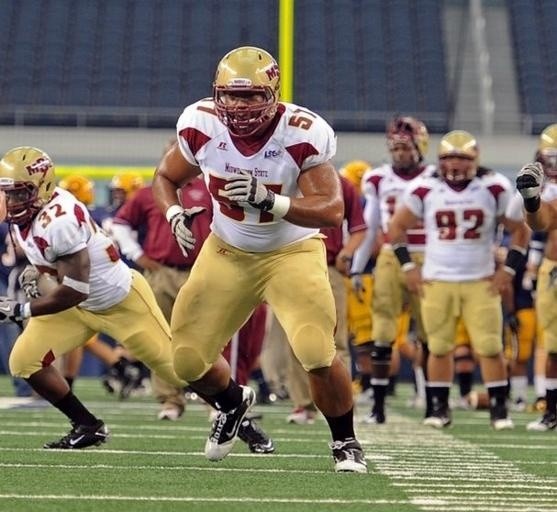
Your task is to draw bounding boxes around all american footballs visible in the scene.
[37,272,59,296]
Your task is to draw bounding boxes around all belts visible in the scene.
[162,261,191,272]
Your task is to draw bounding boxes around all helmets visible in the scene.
[536,123,557,154]
[212,46,281,89]
[0,147,55,186]
[58,175,93,204]
[110,172,144,189]
[385,117,429,140]
[338,160,371,190]
[438,129,480,160]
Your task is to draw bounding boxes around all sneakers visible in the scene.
[353,386,555,431]
[237,415,274,454]
[43,419,107,448]
[102,356,317,425]
[204,383,255,461]
[328,436,367,474]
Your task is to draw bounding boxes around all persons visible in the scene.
[0,118,557,453]
[152,47,368,473]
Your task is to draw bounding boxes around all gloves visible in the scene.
[350,272,366,303]
[168,205,206,259]
[516,161,544,201]
[0,296,24,322]
[224,169,274,213]
[20,270,40,300]
